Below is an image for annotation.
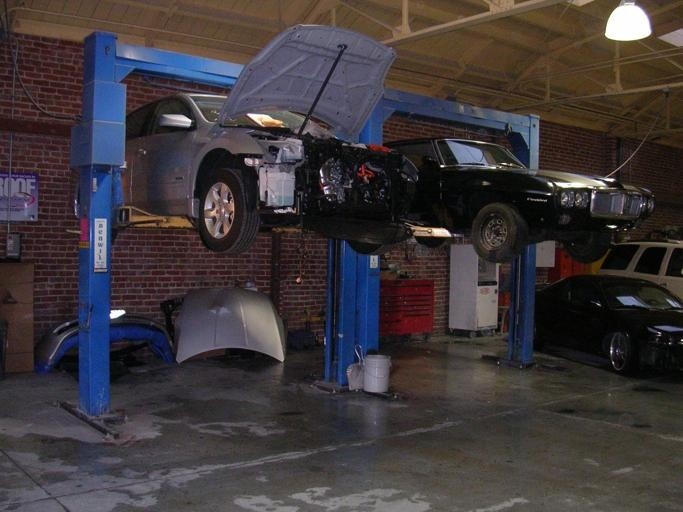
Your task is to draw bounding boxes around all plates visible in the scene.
[0,259,35,373]
[379,278,436,344]
[449,243,498,338]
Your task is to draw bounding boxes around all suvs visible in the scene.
[362,354,391,395]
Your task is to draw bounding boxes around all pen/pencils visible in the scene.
[596,239,682,304]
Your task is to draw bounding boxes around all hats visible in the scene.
[72,23,417,256]
[533,274,682,383]
[345,136,659,262]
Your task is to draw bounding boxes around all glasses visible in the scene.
[604,0,652,41]
